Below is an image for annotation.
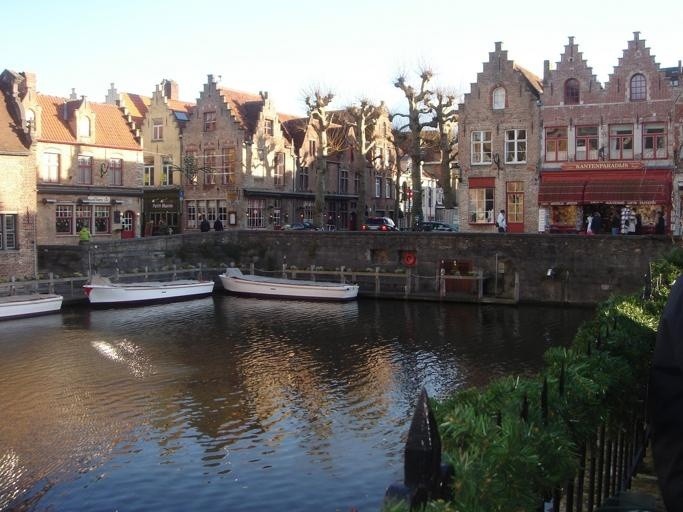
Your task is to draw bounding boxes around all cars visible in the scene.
[281,223,319,230]
[412,221,458,231]
[360,216,399,231]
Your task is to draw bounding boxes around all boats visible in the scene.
[0,295,63,317]
[219,269,359,299]
[82,274,214,304]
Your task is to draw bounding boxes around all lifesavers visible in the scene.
[404,254,415,265]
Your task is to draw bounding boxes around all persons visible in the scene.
[158,220,169,237]
[590,211,600,234]
[607,213,619,235]
[213,216,224,232]
[199,217,210,232]
[584,213,594,234]
[632,214,642,236]
[78,226,91,244]
[496,209,507,233]
[655,211,665,234]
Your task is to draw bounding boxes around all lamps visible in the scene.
[137,212,140,216]
[246,212,341,219]
[120,212,124,217]
